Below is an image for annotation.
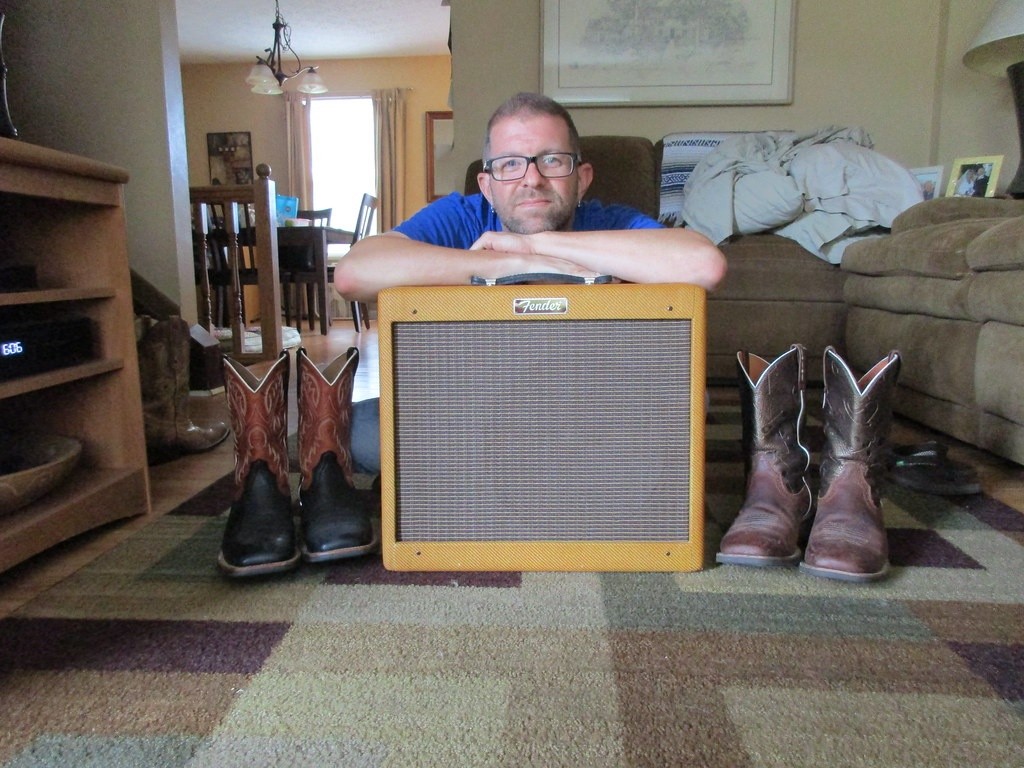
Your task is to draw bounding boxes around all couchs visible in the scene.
[838,195,1024,459]
[967,189,1024,468]
[459,134,841,387]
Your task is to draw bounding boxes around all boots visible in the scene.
[713,348,813,567]
[215,351,301,577]
[798,344,900,582]
[132,314,230,465]
[295,345,378,560]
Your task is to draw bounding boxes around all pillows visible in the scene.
[730,172,804,233]
[797,210,880,252]
[791,138,926,224]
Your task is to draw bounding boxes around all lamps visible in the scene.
[243,0,330,95]
[962,0,1024,200]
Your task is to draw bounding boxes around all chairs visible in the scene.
[206,205,302,332]
[299,206,333,226]
[305,192,384,333]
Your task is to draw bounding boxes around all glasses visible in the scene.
[482,151,580,182]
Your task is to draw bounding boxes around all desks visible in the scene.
[223,224,358,334]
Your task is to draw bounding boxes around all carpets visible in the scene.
[0,383,1024,768]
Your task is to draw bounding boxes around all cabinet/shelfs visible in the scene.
[0,136,154,578]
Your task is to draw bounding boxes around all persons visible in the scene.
[955,169,976,196]
[972,168,990,198]
[923,180,935,200]
[333,92,729,477]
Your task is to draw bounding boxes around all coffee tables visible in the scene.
[375,279,713,575]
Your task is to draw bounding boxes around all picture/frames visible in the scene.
[539,0,799,107]
[424,110,454,204]
[945,156,1003,198]
[205,131,253,183]
[909,165,945,201]
